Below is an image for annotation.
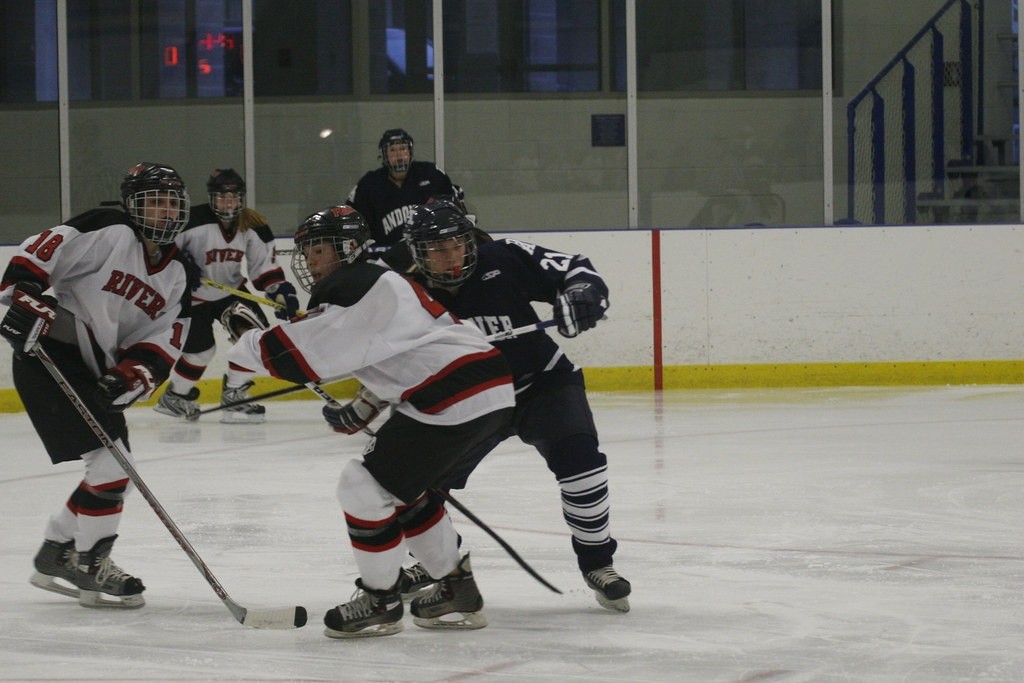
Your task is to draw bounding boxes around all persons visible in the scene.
[320,200,631,615]
[153,168,298,425]
[346,129,465,277]
[227,204,517,637]
[0,162,194,609]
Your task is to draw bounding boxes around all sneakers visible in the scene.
[410,551,487,629]
[29,538,81,600]
[75,534,145,608]
[322,566,406,638]
[219,374,265,424]
[581,564,632,612]
[400,531,463,600]
[153,382,202,422]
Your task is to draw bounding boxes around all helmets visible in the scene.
[122,162,191,246]
[403,199,478,286]
[291,205,371,296]
[206,168,246,223]
[379,128,414,172]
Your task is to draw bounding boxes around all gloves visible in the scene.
[91,358,161,412]
[0,281,58,361]
[265,280,299,321]
[554,280,609,338]
[323,386,390,436]
[221,301,267,344]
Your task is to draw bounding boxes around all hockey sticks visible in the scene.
[198,275,307,320]
[304,380,566,594]
[201,318,557,419]
[28,341,309,627]
